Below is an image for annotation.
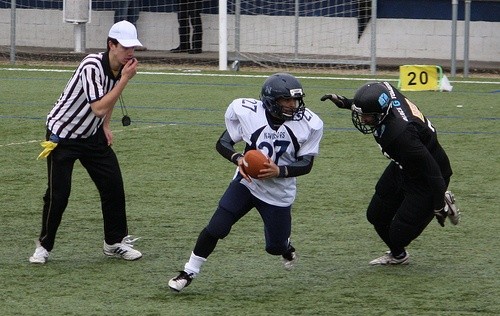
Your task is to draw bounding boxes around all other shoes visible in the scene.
[135,46,146,51]
[170,46,190,53]
[188,48,202,54]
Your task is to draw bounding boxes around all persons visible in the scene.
[321,81,461,266]
[114,0,203,54]
[28,20,142,265]
[168,73,323,292]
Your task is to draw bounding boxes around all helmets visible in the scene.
[261,73,305,121]
[352,83,392,134]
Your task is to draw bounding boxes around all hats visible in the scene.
[108,20,143,47]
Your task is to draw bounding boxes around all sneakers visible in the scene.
[168,271,192,292]
[29,246,49,263]
[282,242,297,272]
[369,251,409,266]
[103,235,142,261]
[443,191,460,226]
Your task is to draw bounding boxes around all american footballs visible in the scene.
[243,150,268,179]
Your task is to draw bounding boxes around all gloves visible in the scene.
[36,134,59,160]
[320,94,351,112]
[434,203,448,227]
